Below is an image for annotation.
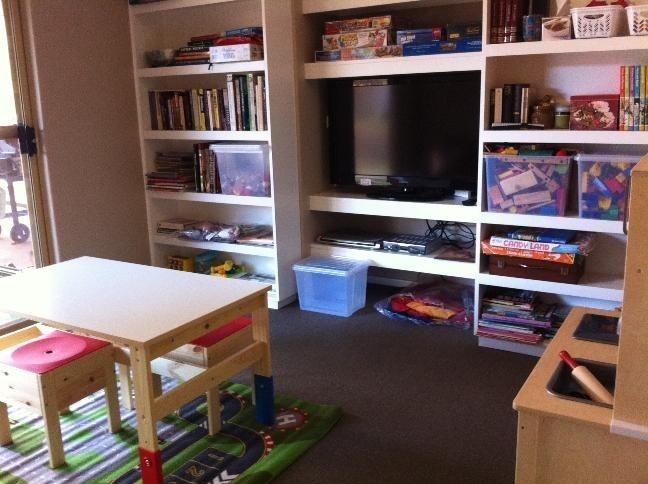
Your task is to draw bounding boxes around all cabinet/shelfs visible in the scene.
[477,0,648,356]
[262,1,486,339]
[131,2,302,312]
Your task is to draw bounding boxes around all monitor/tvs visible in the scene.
[327,72,481,202]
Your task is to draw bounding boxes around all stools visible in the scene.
[161,308,254,437]
[0,330,124,468]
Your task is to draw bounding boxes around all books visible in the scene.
[141,26,274,289]
[478,222,622,346]
[489,81,532,129]
[488,0,550,43]
[618,63,648,130]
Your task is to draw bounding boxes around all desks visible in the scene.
[1,254,277,483]
[542,153,648,356]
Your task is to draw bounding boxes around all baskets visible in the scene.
[571,5,627,38]
[625,5,648,35]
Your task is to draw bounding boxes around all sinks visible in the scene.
[545,358,617,410]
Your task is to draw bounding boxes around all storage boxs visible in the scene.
[483,152,572,217]
[574,153,644,218]
[210,141,270,196]
[291,254,369,318]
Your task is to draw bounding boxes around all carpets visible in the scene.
[2,322,341,484]
